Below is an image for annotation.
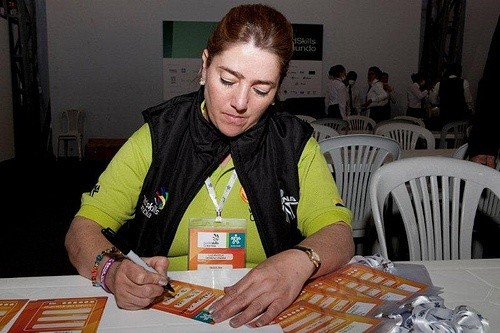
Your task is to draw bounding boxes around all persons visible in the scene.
[325,17,500,170]
[65,5,355,329]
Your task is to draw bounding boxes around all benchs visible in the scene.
[85,137,126,161]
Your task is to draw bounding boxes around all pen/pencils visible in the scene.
[101,227,175,294]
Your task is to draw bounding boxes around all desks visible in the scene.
[325,147,462,210]
[0,258,500,333]
[338,129,468,139]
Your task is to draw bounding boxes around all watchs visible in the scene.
[290,246,322,279]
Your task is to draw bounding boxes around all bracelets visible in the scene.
[91,246,124,294]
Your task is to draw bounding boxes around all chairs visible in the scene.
[296,114,500,259]
[56,108,85,162]
